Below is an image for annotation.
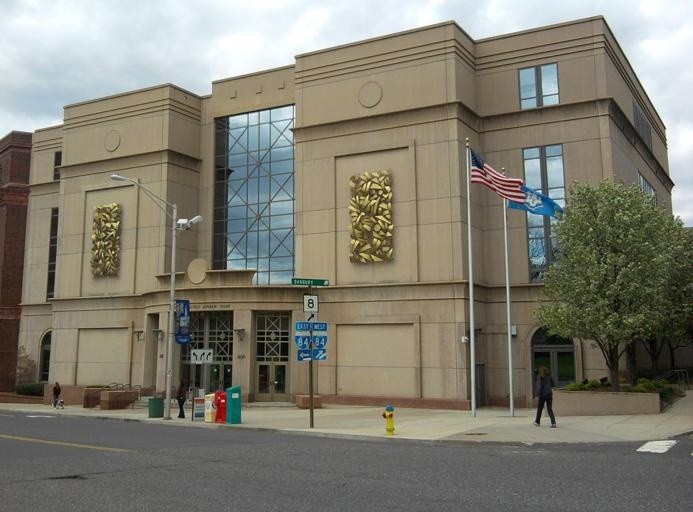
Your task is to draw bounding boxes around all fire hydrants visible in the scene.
[381,403,401,438]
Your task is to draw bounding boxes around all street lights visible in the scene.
[106,171,205,423]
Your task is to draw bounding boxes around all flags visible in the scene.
[507,186,564,220]
[469,149,526,204]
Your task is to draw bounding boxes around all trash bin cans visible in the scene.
[148,398,164,418]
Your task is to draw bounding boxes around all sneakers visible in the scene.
[533,421,556,429]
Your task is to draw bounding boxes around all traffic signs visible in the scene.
[291,278,330,288]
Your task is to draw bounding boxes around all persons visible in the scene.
[55,394,64,410]
[532,366,556,428]
[176,384,186,419]
[52,380,61,408]
